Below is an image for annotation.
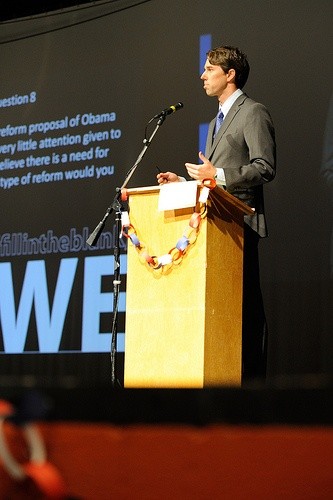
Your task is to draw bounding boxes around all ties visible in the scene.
[214,111,224,135]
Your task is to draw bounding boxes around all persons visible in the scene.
[155,45,279,382]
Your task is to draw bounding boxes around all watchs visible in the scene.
[214,173,218,182]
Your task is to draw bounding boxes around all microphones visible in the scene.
[154,101,184,118]
